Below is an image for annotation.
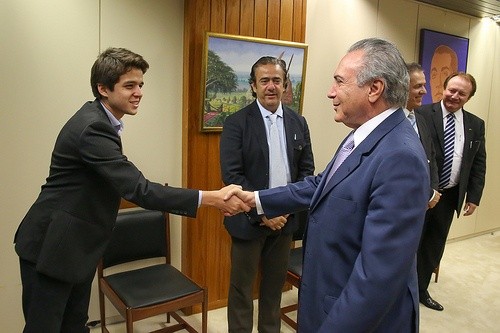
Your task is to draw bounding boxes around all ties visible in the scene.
[408,112,419,140]
[268,114,287,189]
[439,113,454,190]
[319,133,353,194]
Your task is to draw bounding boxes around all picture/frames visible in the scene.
[418,27,470,106]
[197,31,310,134]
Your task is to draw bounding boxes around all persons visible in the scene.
[403,62,443,211]
[13,46,251,333]
[415,72,486,311]
[219,38,431,333]
[219,56,316,333]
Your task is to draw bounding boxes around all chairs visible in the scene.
[97,198,210,333]
[279,206,305,333]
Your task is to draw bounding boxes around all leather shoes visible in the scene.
[418,291,444,311]
